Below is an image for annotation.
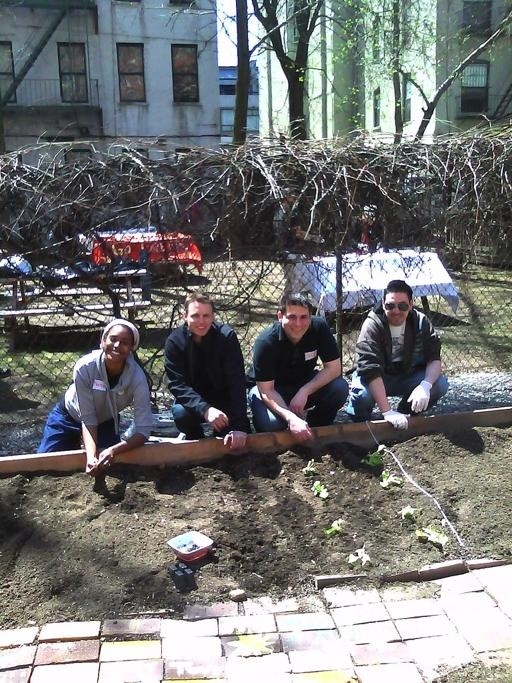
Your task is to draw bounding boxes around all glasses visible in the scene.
[384,302,410,311]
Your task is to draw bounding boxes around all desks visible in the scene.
[1,264,147,325]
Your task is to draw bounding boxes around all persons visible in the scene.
[349,203,385,255]
[162,289,250,451]
[247,293,349,443]
[270,190,325,310]
[38,317,153,476]
[348,280,447,431]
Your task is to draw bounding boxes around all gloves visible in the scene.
[381,409,410,431]
[407,380,432,413]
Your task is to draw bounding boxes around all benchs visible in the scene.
[1,287,152,349]
